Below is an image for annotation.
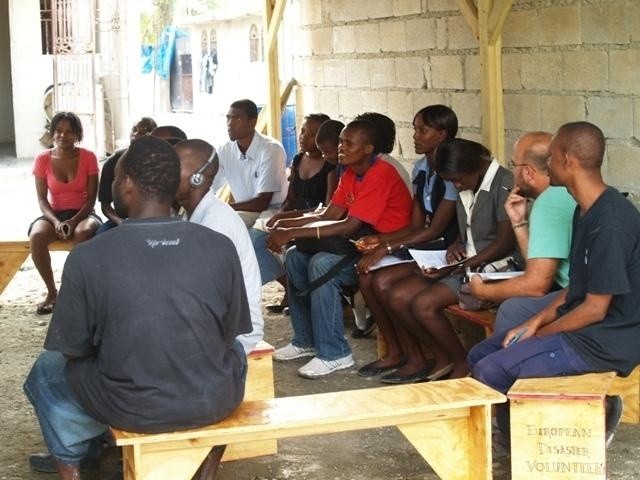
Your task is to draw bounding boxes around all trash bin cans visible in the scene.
[254,105,298,170]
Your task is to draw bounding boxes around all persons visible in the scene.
[461,131,580,337]
[461,119,640,453]
[22,98,527,480]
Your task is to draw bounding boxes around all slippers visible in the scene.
[606,394,624,450]
[36,301,56,315]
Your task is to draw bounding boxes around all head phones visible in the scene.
[189,148,218,187]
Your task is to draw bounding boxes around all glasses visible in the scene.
[507,160,537,172]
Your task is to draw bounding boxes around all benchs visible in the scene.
[111,377,507,480]
[377,302,497,363]
[507,363,640,480]
[0,239,76,296]
[221,339,279,463]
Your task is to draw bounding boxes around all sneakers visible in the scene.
[282,303,291,316]
[263,302,283,314]
[350,324,366,339]
[297,351,355,379]
[363,312,378,337]
[271,341,318,362]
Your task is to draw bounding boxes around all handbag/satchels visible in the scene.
[457,254,524,314]
[387,235,446,262]
[293,218,367,256]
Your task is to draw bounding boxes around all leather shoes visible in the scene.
[27,451,88,474]
[379,360,429,383]
[356,352,409,378]
[78,456,103,474]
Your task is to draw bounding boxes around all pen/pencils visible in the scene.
[501,186,531,203]
[507,328,528,346]
[448,243,468,257]
[348,239,367,248]
